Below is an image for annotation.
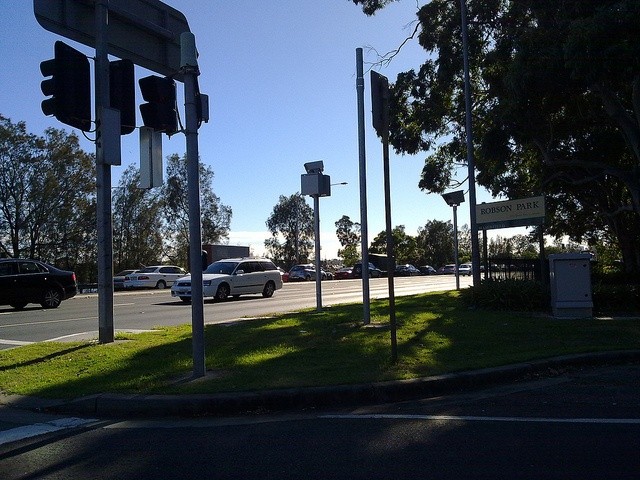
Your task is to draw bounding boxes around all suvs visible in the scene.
[353,263,384,277]
[443,264,456,274]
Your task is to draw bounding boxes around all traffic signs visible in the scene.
[477,195,545,229]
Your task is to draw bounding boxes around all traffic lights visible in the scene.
[138,75,178,134]
[40,41,91,132]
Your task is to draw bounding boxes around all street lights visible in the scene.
[295,182,347,264]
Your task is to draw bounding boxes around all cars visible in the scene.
[0,259,78,308]
[420,266,437,275]
[454,264,472,276]
[123,266,191,289]
[171,257,283,302]
[334,267,353,279]
[393,264,420,276]
[114,269,140,289]
[288,264,328,281]
